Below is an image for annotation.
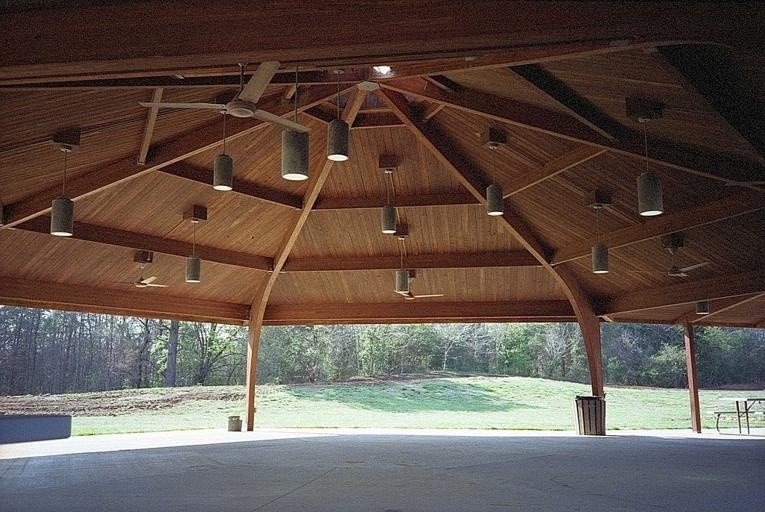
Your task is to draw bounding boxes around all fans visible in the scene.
[135,63,312,135]
[101,251,168,290]
[631,234,710,279]
[393,289,444,301]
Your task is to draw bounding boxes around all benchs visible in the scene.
[714,409,765,432]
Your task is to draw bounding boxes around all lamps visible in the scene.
[182,204,210,286]
[588,94,667,276]
[211,62,349,196]
[46,127,81,240]
[375,151,414,295]
[478,126,511,217]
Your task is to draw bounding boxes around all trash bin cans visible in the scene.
[575,395,606,435]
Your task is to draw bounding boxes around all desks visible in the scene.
[720,398,765,433]
